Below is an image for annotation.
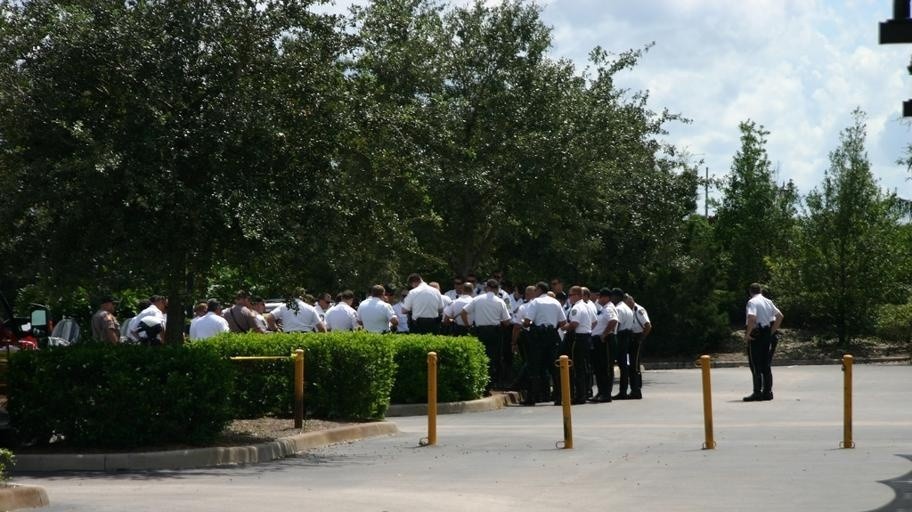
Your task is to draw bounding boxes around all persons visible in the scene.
[742,283,784,401]
[761,289,778,400]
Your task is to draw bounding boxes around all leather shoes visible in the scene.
[516,390,643,407]
[743,390,761,402]
[760,390,777,400]
